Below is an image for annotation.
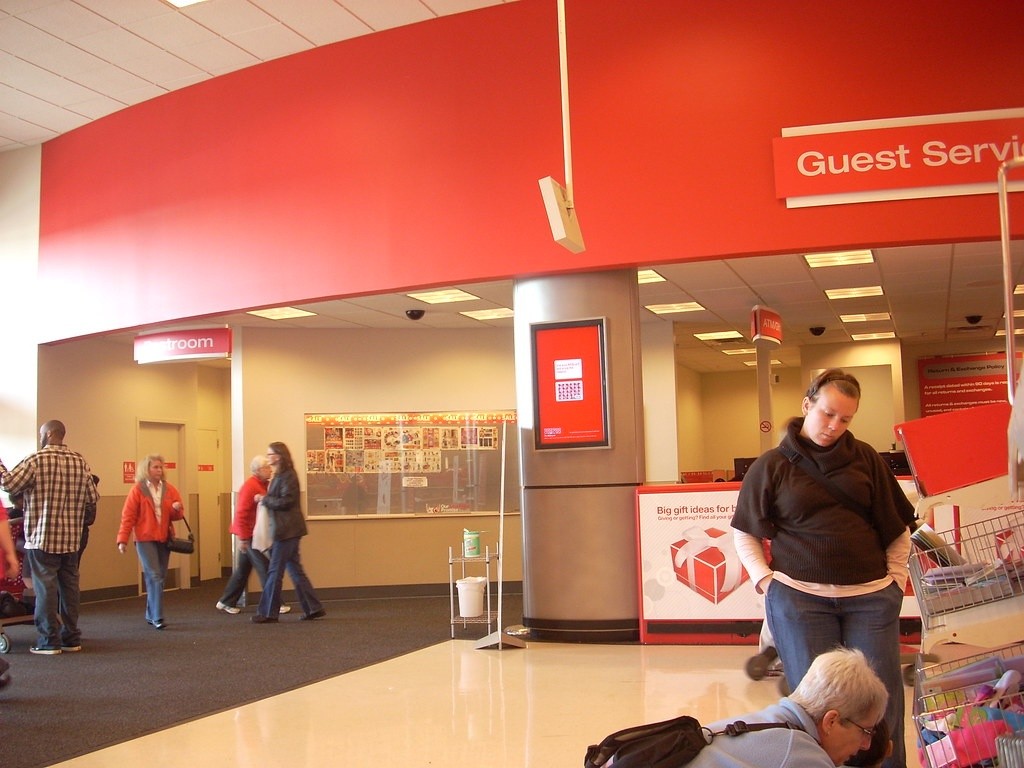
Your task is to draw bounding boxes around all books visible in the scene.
[910,523,1014,614]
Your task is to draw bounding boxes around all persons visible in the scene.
[216,442,326,624]
[680,646,893,768]
[117,455,184,628]
[0,419,101,686]
[730,367,919,768]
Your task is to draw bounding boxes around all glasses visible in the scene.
[846,718,878,737]
[267,453,277,456]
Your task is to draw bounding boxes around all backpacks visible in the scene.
[583,714,820,768]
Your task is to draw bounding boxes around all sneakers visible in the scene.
[30,645,62,654]
[61,643,82,651]
[216,601,241,614]
[279,606,290,614]
[299,609,325,620]
[250,614,278,623]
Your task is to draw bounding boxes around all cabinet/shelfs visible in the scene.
[448,542,503,640]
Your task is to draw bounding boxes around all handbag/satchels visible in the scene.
[167,513,195,554]
[252,500,275,552]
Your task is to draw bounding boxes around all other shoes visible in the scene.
[148,618,167,629]
